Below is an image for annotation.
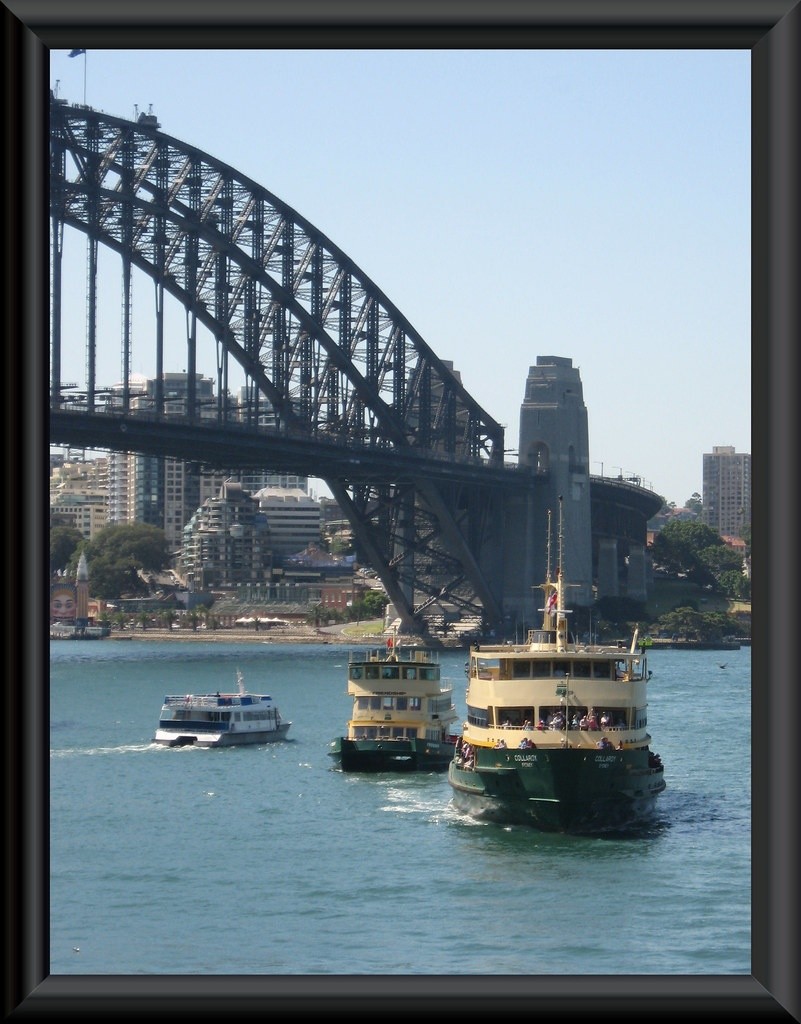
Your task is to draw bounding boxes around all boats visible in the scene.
[149,668,292,748]
[327,624,460,773]
[446,492,668,825]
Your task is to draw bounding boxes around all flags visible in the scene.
[67,49,86,58]
[546,568,561,615]
[385,638,393,648]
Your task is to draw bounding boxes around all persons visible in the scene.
[491,739,507,749]
[502,719,513,730]
[517,738,537,750]
[522,720,532,731]
[452,737,476,773]
[549,711,610,731]
[556,664,566,677]
[596,737,623,750]
